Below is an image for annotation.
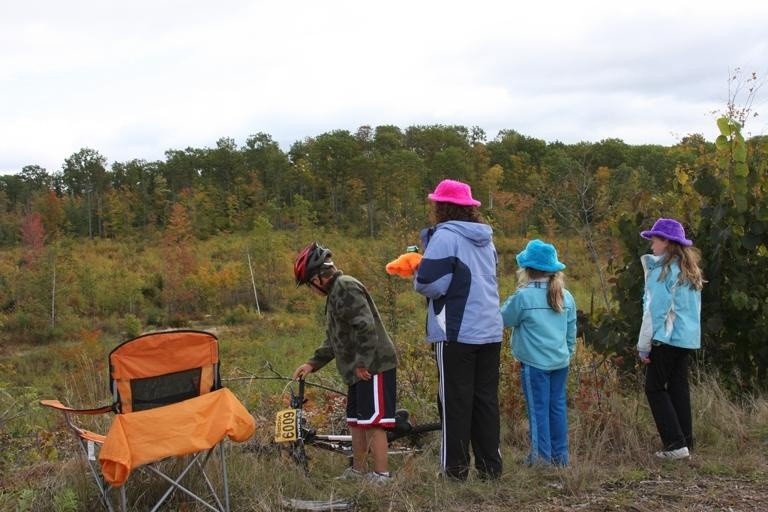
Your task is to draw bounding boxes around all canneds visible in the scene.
[407,246,418,253]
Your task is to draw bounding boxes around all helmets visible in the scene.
[292,240,334,289]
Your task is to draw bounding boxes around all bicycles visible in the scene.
[259,359,442,481]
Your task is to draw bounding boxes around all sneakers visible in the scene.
[332,465,364,481]
[652,446,692,462]
[362,470,394,484]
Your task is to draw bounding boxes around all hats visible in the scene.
[427,179,482,208]
[639,216,694,247]
[515,238,566,272]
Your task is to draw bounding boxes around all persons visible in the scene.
[407,178,504,482]
[637,218,702,460]
[292,242,402,484]
[497,239,577,468]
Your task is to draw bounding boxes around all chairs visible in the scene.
[37,327,257,511]
